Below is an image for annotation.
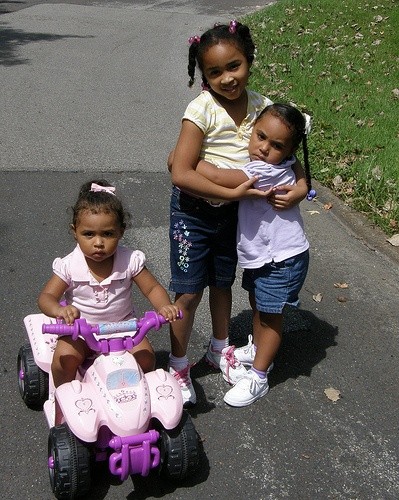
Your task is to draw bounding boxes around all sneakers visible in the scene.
[167,363,196,407]
[205,342,247,385]
[223,370,269,407]
[234,334,274,371]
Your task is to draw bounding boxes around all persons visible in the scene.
[166,102,310,407]
[37,179,180,427]
[167,18,309,408]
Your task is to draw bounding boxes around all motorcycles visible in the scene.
[15,297,203,500]
